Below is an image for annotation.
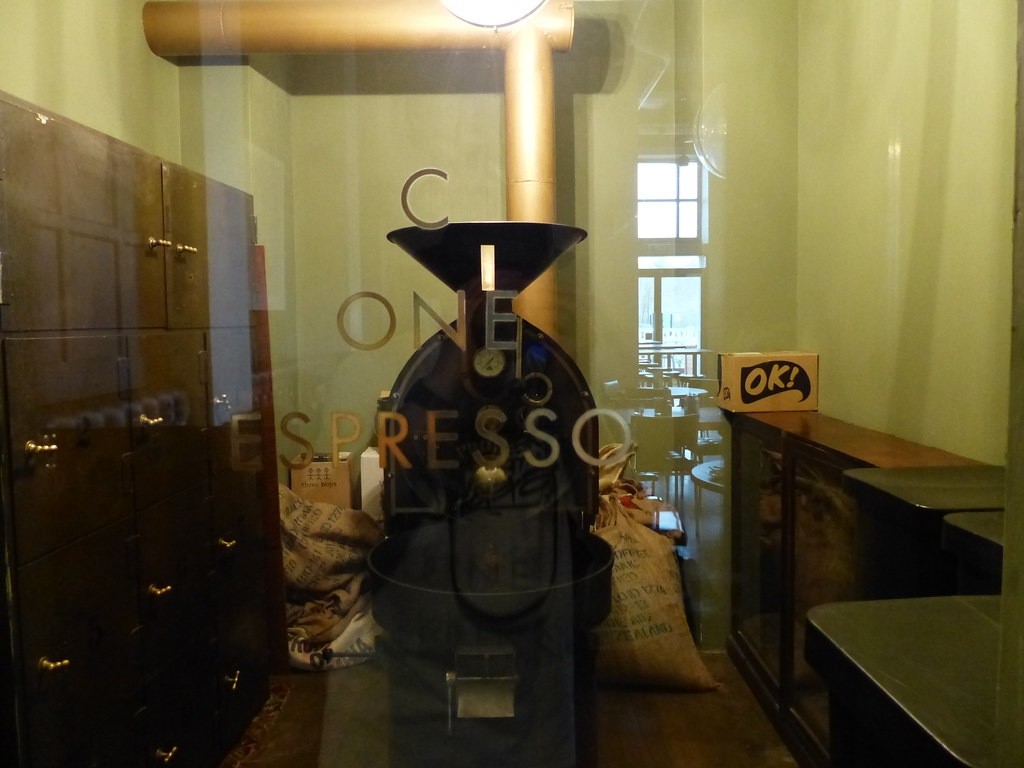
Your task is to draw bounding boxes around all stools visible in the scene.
[638,360,705,388]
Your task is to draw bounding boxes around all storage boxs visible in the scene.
[717,352,819,411]
[360,444,385,527]
[290,452,353,509]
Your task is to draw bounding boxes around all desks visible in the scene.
[690,460,723,541]
[669,387,708,398]
[639,339,712,375]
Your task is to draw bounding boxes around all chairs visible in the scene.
[596,386,700,540]
[697,438,723,466]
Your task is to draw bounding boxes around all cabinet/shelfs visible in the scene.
[1,333,130,563]
[68,598,293,767]
[844,464,1003,596]
[192,445,282,607]
[0,89,255,332]
[130,330,215,518]
[723,410,971,766]
[0,515,151,768]
[205,329,261,479]
[940,504,1024,597]
[804,595,1023,766]
[108,492,219,705]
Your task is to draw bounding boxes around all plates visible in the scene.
[708,466,725,472]
[706,471,720,477]
